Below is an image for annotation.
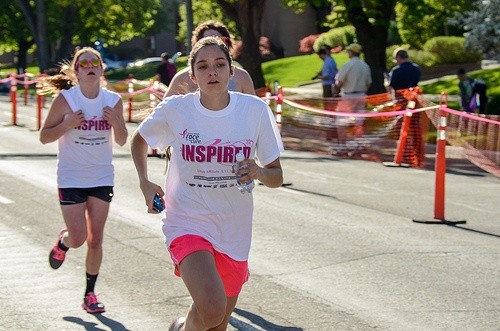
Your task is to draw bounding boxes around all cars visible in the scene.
[126,57,163,69]
[103,59,126,75]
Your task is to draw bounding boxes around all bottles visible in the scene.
[233,152,255,193]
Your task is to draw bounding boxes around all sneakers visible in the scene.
[82,292,105,313]
[49,228,71,270]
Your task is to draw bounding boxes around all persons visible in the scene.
[162,21,258,103]
[132,37,287,331]
[384,50,426,164]
[331,44,374,161]
[33,46,128,316]
[318,47,339,115]
[157,52,178,89]
[457,68,480,117]
[323,45,332,57]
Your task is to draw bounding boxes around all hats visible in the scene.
[345,43,361,53]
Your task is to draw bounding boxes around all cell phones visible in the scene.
[154,194,165,212]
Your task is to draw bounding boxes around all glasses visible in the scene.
[75,58,102,71]
